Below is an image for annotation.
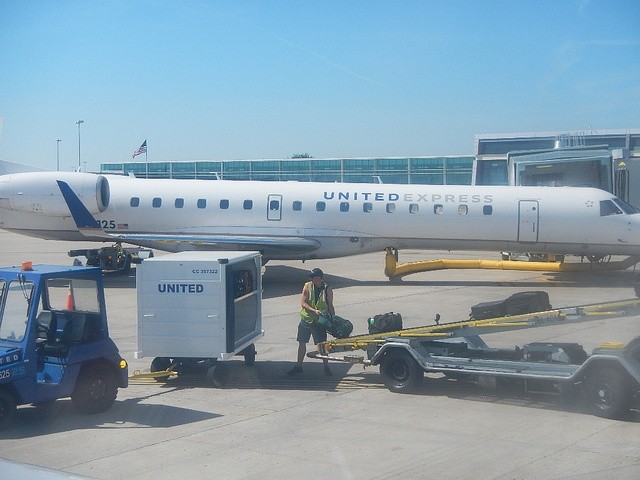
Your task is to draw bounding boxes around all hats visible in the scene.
[307,268,323,277]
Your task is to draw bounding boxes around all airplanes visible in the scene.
[1,171,640,277]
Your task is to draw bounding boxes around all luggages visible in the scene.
[507,292,552,315]
[470,301,507,319]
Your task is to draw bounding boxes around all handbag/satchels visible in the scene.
[367,312,402,334]
[316,312,353,339]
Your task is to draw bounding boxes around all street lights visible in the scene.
[55,139,62,171]
[75,121,84,172]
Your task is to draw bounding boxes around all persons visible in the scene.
[287,268,335,377]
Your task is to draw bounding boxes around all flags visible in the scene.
[132,140,146,159]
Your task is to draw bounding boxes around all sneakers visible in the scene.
[288,365,302,375]
[324,367,331,375]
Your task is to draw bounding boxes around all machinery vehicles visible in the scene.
[305,299,640,423]
[0,260,129,431]
